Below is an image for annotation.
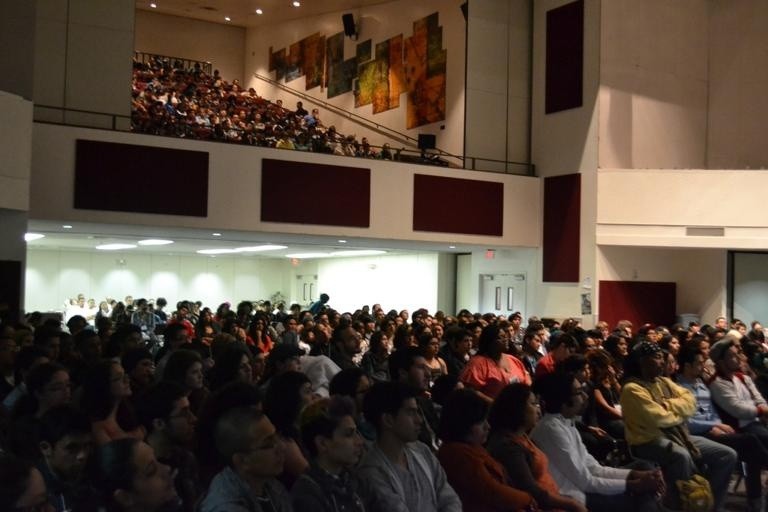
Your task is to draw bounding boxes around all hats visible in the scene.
[638,340,661,358]
[263,342,307,366]
[709,339,733,361]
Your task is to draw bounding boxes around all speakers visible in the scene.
[341,13,355,37]
[418,134,436,148]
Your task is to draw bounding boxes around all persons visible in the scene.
[131,58,392,160]
[0,294,768,512]
